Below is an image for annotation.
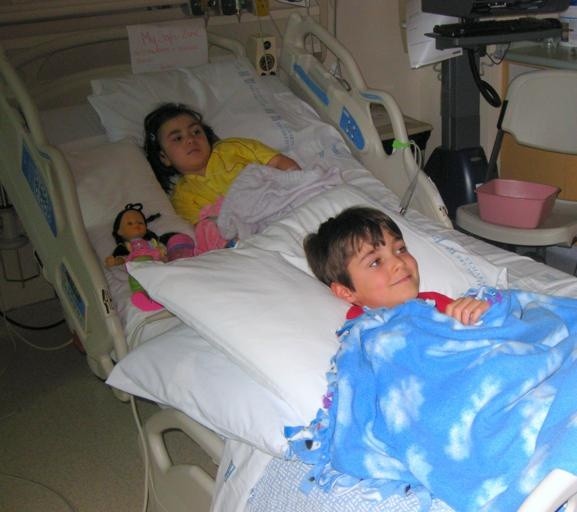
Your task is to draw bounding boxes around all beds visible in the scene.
[0,0,577,512]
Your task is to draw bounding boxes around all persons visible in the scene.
[297,204,495,328]
[140,101,303,233]
[100,199,166,312]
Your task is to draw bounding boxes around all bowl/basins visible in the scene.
[473,177,560,230]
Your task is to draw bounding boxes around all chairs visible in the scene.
[455,70,577,262]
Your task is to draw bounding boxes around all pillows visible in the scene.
[105,322,309,460]
[127,182,477,429]
[87,56,302,154]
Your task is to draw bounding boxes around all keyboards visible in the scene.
[433,17,564,37]
